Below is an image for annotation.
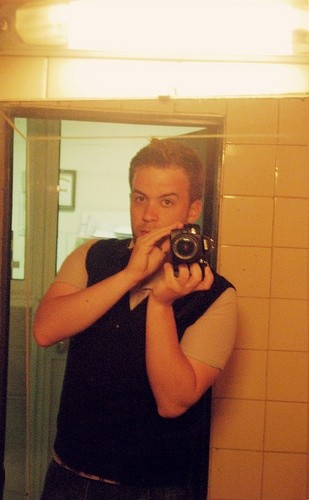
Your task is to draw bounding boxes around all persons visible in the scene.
[34,138,239,500]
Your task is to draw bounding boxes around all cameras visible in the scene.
[170,223,213,275]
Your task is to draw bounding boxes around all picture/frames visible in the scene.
[59,169,77,211]
